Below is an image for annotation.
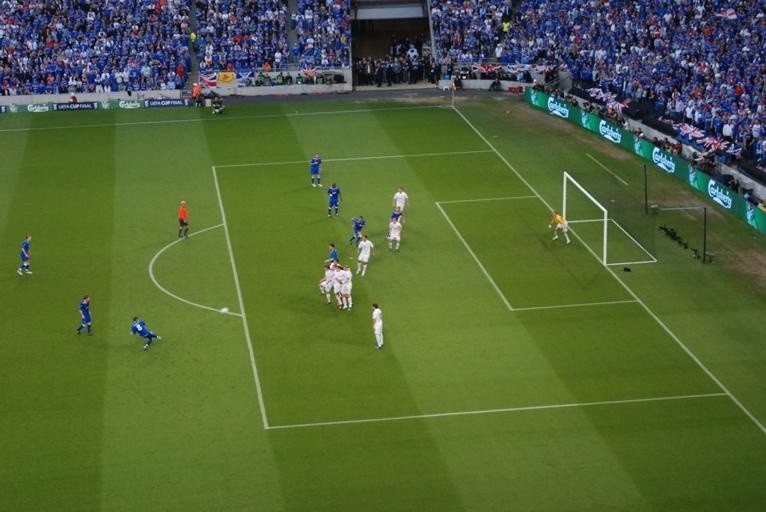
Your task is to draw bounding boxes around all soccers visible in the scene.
[220,308,228,314]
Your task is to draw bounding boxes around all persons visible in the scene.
[389,206,406,224]
[177,200,191,238]
[129,316,163,350]
[309,152,324,188]
[76,294,96,336]
[318,214,376,311]
[2,0,362,114]
[392,186,410,212]
[431,0,765,213]
[386,217,402,253]
[16,233,33,276]
[546,211,572,245]
[370,303,384,350]
[327,182,344,218]
[362,30,430,88]
